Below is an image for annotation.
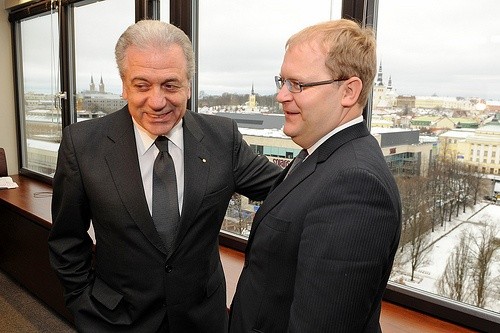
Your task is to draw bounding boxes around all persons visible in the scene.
[226,20,402,333]
[48,20,283,333]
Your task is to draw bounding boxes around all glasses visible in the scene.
[275,75,351,93]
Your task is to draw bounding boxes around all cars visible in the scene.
[435,200,444,208]
[457,194,467,203]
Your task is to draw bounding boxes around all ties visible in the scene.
[282,149,309,181]
[152,135,181,255]
